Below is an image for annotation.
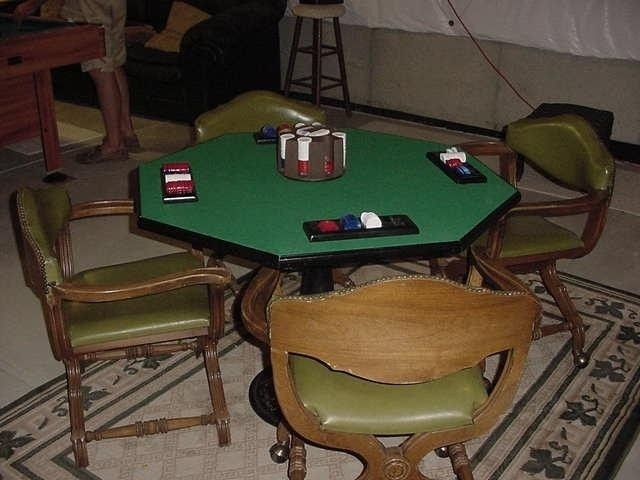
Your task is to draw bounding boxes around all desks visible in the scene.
[136,128,521,428]
[0,12,106,174]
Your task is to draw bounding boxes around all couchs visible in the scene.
[51,0,287,127]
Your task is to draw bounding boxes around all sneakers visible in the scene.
[76,132,140,165]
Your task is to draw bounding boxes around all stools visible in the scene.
[283,4,352,118]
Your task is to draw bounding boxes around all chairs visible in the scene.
[194,90,356,298]
[427,113,616,375]
[267,273,543,480]
[9,190,233,469]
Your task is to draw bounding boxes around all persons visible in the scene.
[56,1,142,165]
[1,1,37,36]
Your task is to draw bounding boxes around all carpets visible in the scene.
[0,249,640,480]
